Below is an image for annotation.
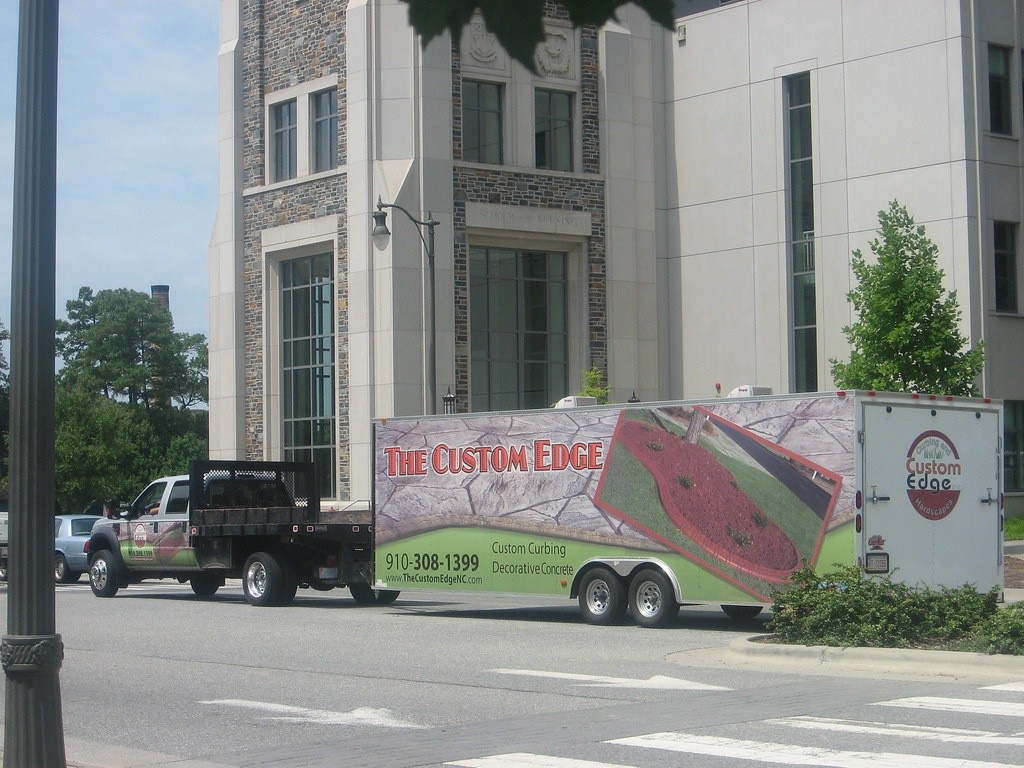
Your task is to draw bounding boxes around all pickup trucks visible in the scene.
[88,458,401,606]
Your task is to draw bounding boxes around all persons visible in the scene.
[150,499,161,514]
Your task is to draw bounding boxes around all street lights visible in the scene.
[371,199,441,416]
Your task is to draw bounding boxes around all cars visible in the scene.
[54,513,108,584]
